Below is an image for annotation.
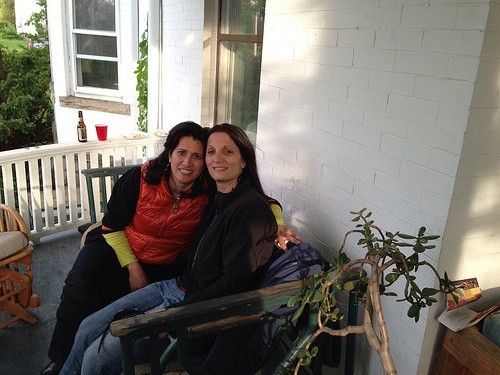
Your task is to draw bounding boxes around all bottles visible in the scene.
[77,111,87,142]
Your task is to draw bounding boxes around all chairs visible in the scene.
[0,202,40,329]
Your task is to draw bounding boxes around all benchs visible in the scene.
[82,165,367,375]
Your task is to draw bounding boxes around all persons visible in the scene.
[39,120,301,375]
[60,123,279,375]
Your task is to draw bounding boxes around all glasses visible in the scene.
[98,308,145,353]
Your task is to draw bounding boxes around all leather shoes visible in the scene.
[41,360,60,375]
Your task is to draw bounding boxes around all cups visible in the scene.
[95,124,108,141]
